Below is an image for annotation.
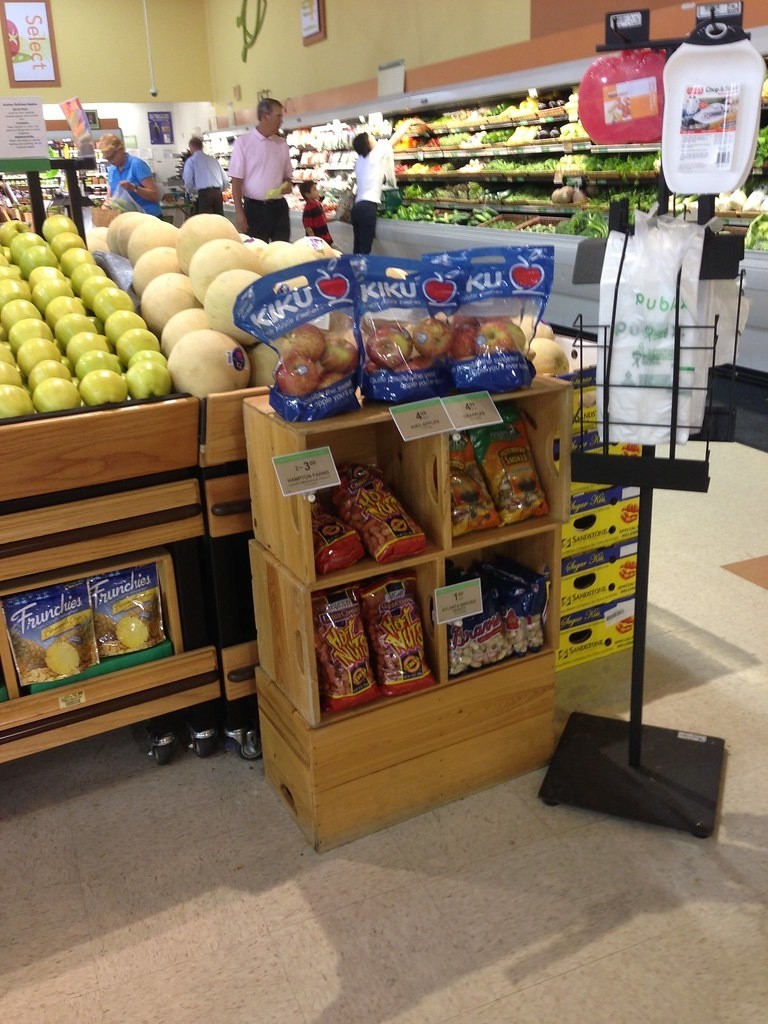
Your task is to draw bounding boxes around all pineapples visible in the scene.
[4,605,163,685]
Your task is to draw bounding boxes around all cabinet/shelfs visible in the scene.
[242,374,574,853]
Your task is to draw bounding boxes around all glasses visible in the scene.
[102,151,116,160]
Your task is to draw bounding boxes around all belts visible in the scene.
[198,186,220,193]
[243,196,287,207]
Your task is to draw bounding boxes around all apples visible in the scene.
[274,313,533,422]
[1,214,171,418]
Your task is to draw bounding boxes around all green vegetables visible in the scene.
[385,100,768,253]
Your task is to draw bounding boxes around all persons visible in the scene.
[299,179,333,248]
[182,138,229,215]
[228,98,294,242]
[351,119,411,253]
[99,134,163,220]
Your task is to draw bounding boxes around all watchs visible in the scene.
[133,184,139,193]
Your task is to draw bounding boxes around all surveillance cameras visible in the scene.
[151,87,158,96]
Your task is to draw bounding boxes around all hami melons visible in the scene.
[106,211,346,397]
[516,313,569,378]
